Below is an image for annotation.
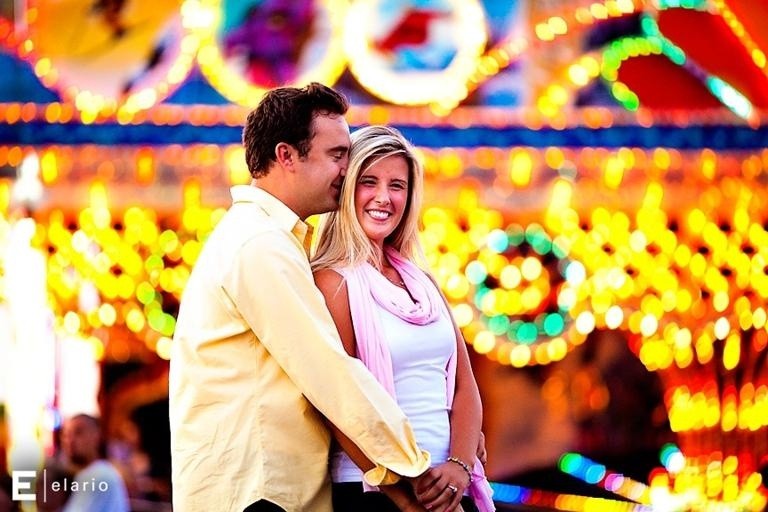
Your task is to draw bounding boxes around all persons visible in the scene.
[108,420,171,511]
[58,414,130,511]
[167,82,468,512]
[1,403,24,511]
[34,423,75,511]
[306,124,498,512]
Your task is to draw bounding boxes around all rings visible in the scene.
[449,486,459,493]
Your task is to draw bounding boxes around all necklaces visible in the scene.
[367,258,407,287]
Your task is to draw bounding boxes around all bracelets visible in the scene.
[447,456,476,485]
